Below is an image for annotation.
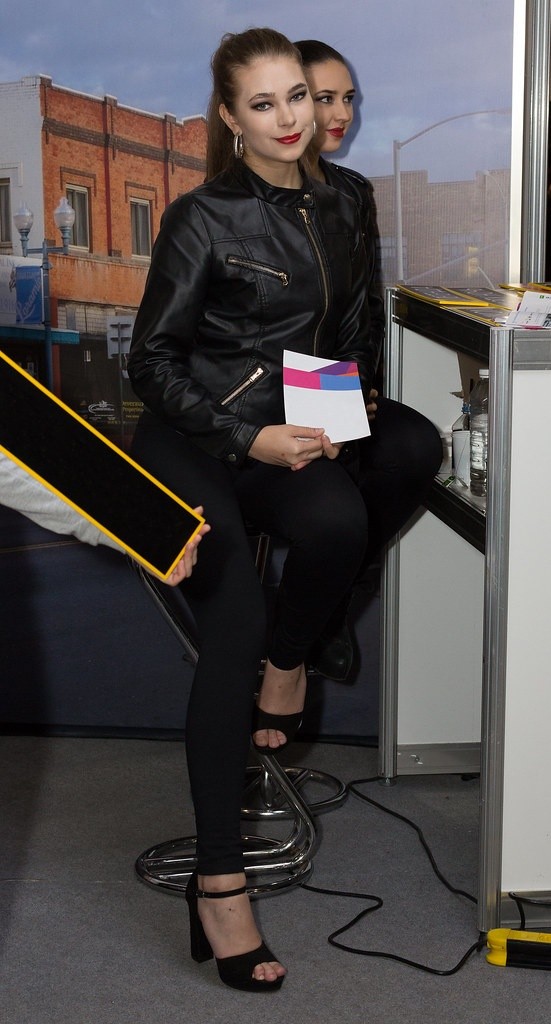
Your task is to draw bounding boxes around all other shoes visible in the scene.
[308,617,353,681]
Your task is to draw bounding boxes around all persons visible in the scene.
[292,38,443,683]
[122,26,379,996]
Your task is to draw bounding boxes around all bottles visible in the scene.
[448,398,471,484]
[470,367,490,498]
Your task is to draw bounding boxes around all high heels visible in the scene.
[254,701,307,756]
[184,872,284,991]
